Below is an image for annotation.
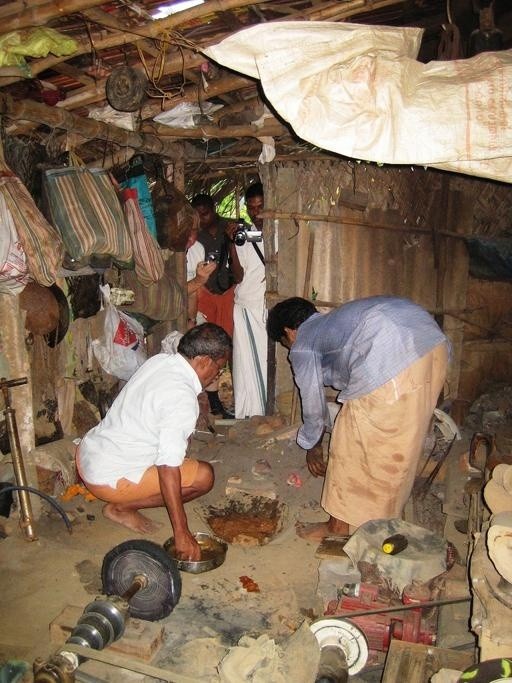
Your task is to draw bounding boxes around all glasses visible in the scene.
[211,357,226,377]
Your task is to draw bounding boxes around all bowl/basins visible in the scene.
[161,533,228,575]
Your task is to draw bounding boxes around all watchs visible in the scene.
[187,317,196,324]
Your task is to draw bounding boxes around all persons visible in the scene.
[75,323,233,562]
[266,295,452,540]
[191,194,252,419]
[225,183,268,419]
[186,208,217,330]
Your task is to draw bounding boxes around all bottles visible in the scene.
[401,579,431,608]
[383,533,409,556]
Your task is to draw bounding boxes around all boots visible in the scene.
[205,389,235,420]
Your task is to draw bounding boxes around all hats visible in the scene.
[485,524,512,585]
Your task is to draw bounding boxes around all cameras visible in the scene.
[206,251,221,265]
[233,218,262,246]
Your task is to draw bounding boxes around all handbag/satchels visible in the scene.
[204,264,236,297]
[0,151,200,321]
